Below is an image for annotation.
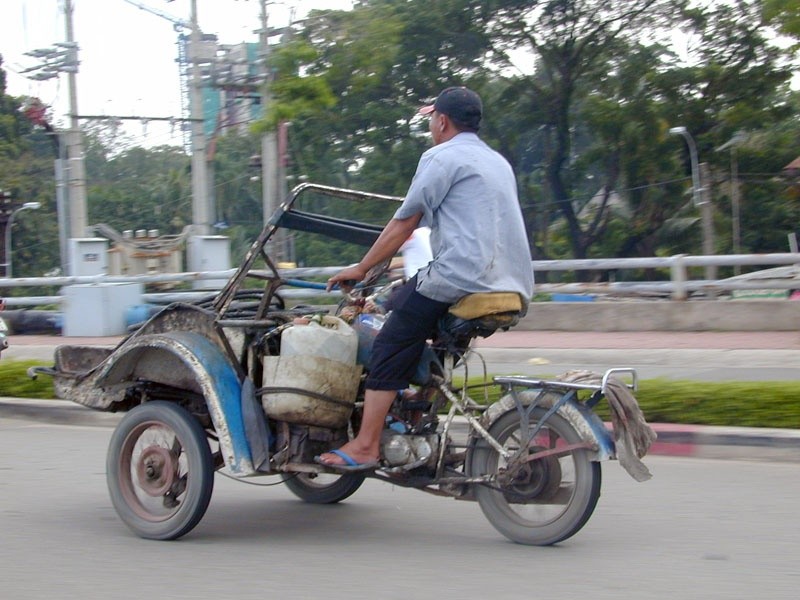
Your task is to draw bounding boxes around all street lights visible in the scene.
[668,125,717,299]
[5,202,40,279]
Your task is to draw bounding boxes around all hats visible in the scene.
[420,86,482,122]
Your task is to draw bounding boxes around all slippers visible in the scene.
[314,449,378,471]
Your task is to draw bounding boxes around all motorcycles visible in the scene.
[25,181,638,546]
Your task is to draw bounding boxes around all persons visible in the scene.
[314,86,534,472]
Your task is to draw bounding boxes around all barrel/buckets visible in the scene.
[261,355,366,428]
[280,315,358,367]
